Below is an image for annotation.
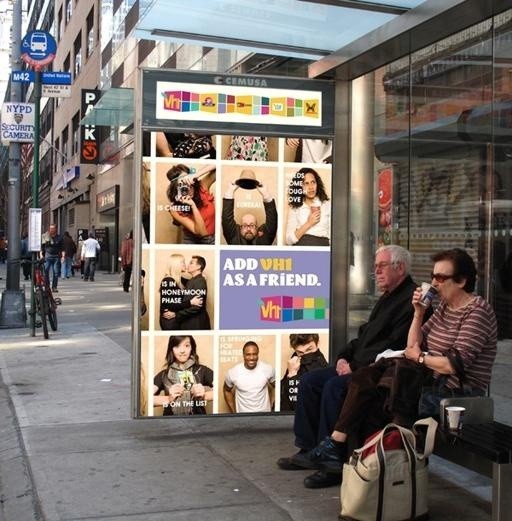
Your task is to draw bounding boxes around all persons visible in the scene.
[120,230,133,293]
[296,247,498,491]
[279,334,327,411]
[275,245,437,472]
[155,336,214,416]
[159,254,211,331]
[0,222,103,292]
[223,341,275,413]
[220,178,278,245]
[285,168,328,246]
[222,135,269,161]
[286,137,328,163]
[166,164,216,244]
[156,132,216,159]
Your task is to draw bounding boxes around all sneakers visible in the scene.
[290,436,344,471]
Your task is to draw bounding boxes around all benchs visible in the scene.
[346,420,512,521]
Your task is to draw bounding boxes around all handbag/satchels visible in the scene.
[340,424,438,519]
[419,381,481,436]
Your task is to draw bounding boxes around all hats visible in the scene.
[235,169,262,190]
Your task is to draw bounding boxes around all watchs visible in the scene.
[416,352,428,366]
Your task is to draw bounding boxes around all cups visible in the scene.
[446,406,466,432]
[417,281,439,308]
[311,205,320,212]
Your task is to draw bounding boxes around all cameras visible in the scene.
[176,182,191,197]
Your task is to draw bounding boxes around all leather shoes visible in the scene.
[304,471,341,489]
[278,449,312,471]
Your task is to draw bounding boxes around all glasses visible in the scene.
[431,272,460,282]
[375,262,399,269]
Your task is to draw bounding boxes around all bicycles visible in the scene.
[9,256,61,339]
